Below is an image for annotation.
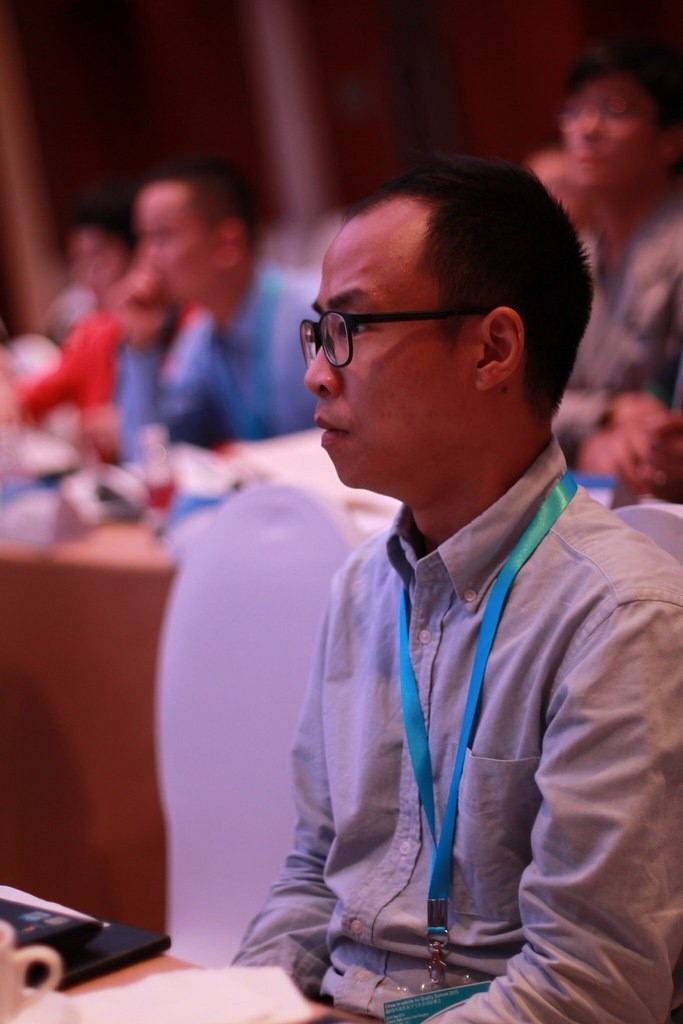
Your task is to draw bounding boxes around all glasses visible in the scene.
[299,307,490,370]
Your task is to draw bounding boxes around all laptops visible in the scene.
[0,896,104,967]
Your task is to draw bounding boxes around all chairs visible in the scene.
[153,480,359,967]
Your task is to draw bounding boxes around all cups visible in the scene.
[0,918,62,1024]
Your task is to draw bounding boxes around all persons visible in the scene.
[230,160,683,1024]
[531,36,683,507]
[0,167,321,495]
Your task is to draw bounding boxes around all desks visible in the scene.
[56,953,381,1024]
[1,510,168,932]
